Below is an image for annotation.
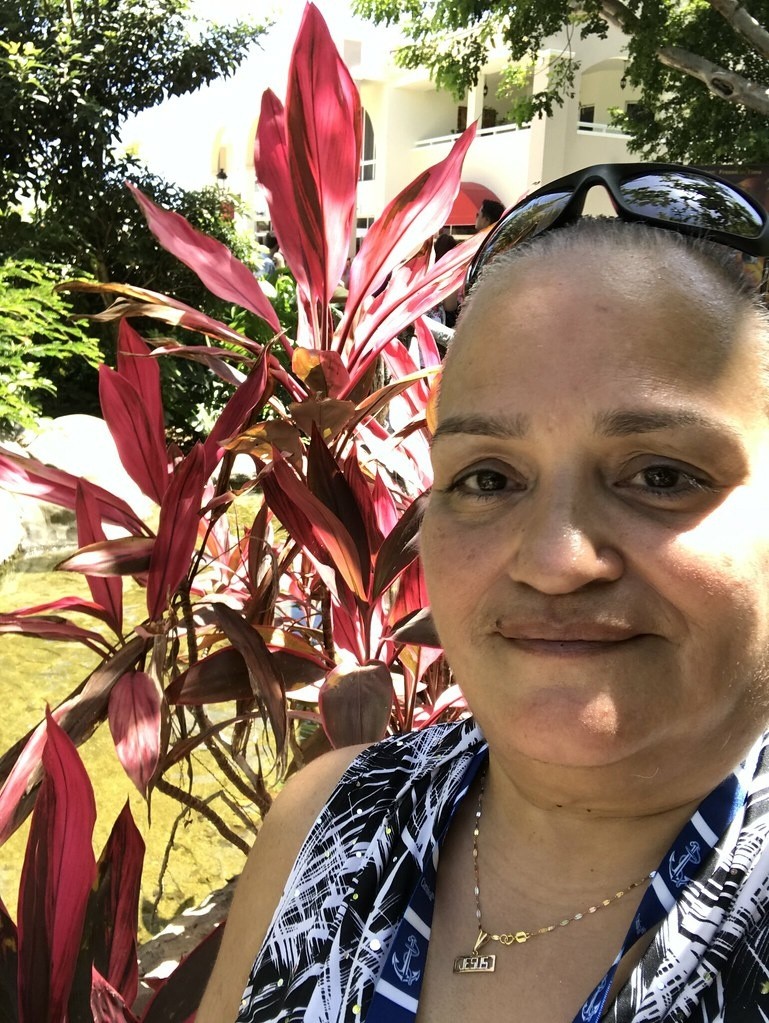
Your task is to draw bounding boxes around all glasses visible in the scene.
[460,165,769,281]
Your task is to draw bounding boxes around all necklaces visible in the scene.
[452,768,661,974]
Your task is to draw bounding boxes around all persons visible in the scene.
[191,214,768,1022]
